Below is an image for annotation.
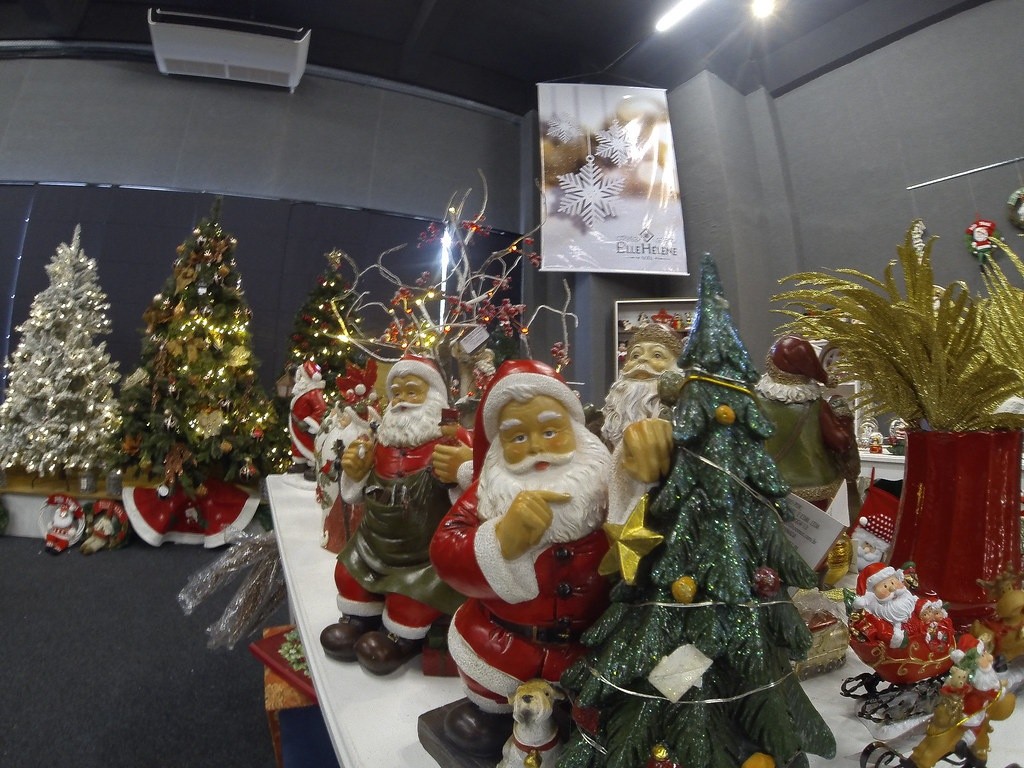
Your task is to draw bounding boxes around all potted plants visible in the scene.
[763,220,1023,659]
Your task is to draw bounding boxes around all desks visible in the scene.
[260,474,1023,768]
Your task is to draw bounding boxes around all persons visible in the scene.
[44,505,115,553]
[288,318,1008,763]
